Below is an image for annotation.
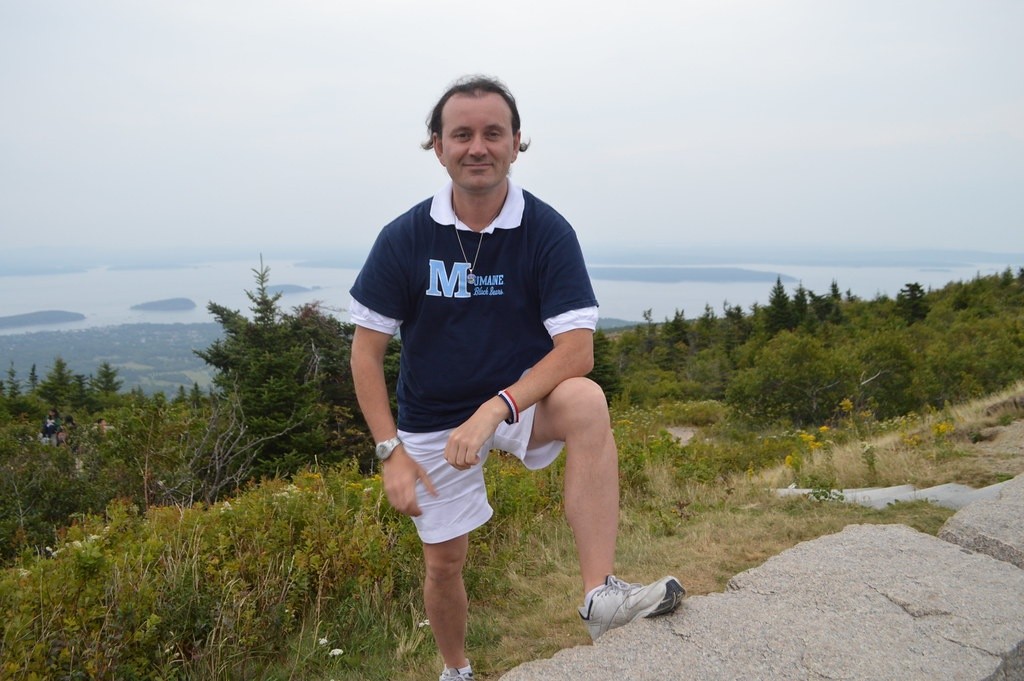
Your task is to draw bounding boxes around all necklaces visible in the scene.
[453,204,502,284]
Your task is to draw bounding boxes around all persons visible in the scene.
[41,407,107,447]
[349,78,687,681]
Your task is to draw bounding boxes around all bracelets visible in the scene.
[497,390,520,424]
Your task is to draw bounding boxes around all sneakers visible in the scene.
[438,658,475,681]
[576,573,687,640]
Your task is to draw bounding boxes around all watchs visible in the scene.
[374,436,402,460]
